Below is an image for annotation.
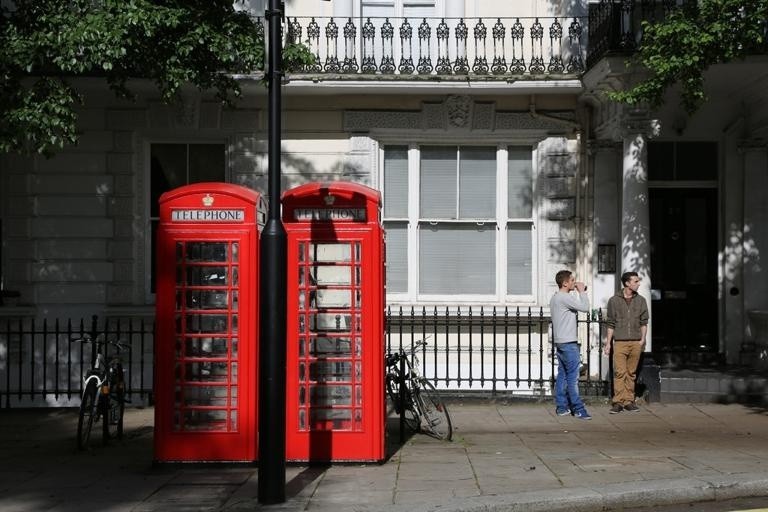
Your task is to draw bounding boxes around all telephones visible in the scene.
[301,270,317,309]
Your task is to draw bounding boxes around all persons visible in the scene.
[548,270,592,419]
[603,271,649,414]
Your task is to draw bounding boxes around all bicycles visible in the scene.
[69,336,133,449]
[384,337,453,440]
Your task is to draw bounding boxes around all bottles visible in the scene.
[574,284,587,291]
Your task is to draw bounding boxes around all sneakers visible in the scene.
[558,409,591,420]
[610,403,640,413]
